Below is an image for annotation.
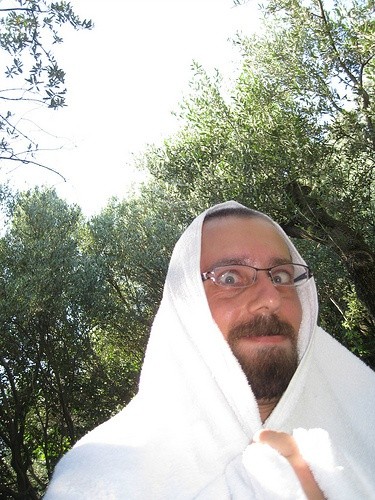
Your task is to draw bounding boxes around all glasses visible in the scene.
[201,263,314,289]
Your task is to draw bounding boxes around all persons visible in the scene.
[40,199,375,500]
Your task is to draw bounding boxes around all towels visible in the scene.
[38,200,374,500]
